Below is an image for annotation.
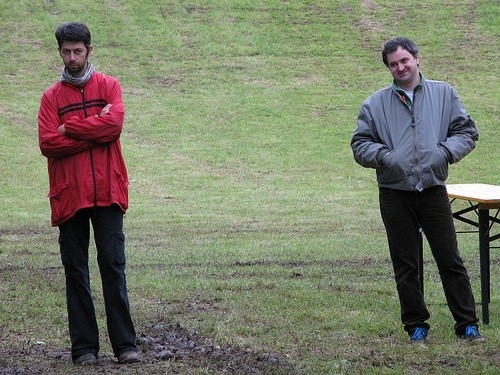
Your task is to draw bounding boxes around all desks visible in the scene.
[419,183,500,325]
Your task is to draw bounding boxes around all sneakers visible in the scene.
[461,326,487,343]
[407,327,428,346]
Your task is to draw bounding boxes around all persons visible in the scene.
[38,23,140,366]
[349,36,486,347]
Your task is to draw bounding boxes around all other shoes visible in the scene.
[117,351,140,364]
[76,353,98,366]
